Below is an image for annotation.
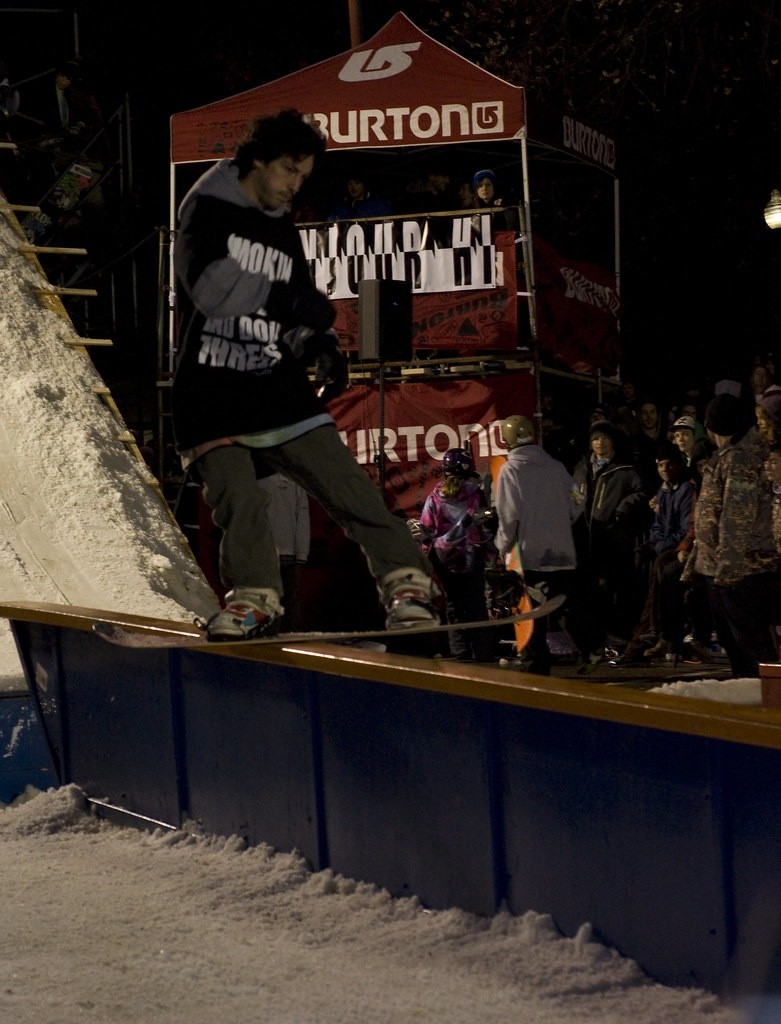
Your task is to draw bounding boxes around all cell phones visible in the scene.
[581,484,585,493]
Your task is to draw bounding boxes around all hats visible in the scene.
[758,395,781,422]
[589,429,611,442]
[704,392,741,436]
[668,416,697,432]
[650,441,678,461]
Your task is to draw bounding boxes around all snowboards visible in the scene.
[90,592,573,654]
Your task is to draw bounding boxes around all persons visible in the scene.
[405,365,781,677]
[170,108,449,643]
[330,166,518,241]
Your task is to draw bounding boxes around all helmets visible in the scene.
[501,415,535,451]
[443,448,472,480]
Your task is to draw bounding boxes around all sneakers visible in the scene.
[205,586,286,642]
[377,568,443,633]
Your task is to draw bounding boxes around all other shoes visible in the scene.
[499,653,552,674]
[575,639,715,679]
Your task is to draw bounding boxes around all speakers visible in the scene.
[358,278,413,363]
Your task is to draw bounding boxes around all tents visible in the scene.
[141,10,620,381]
